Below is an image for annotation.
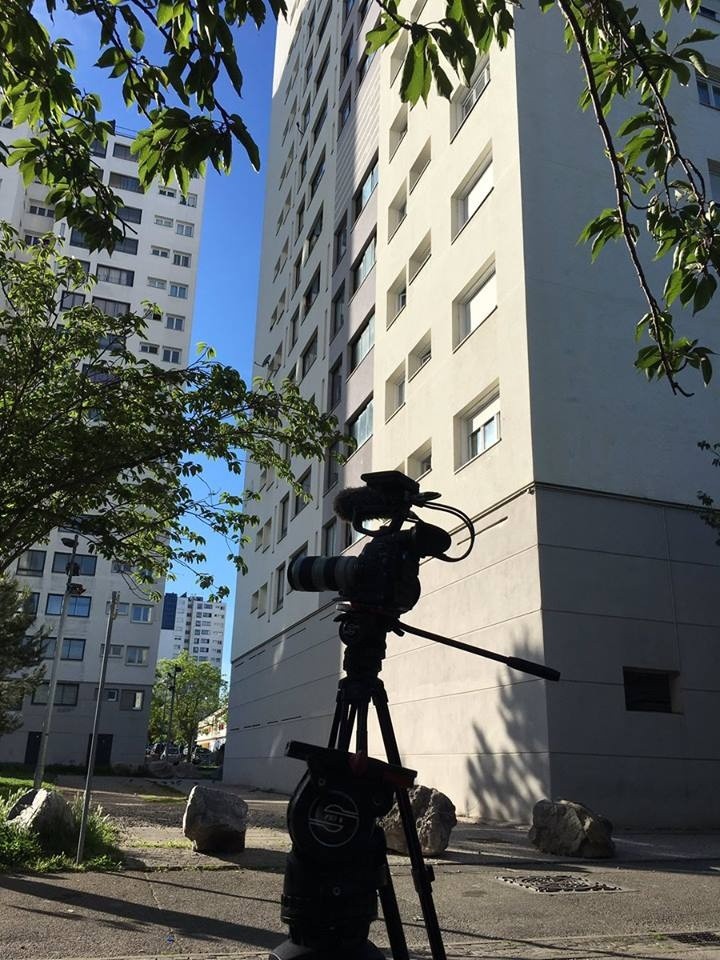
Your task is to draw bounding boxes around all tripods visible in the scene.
[329,610,562,960]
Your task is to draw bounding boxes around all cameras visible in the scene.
[285,470,476,616]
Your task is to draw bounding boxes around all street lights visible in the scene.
[28,529,80,791]
[69,588,121,865]
[165,662,180,758]
